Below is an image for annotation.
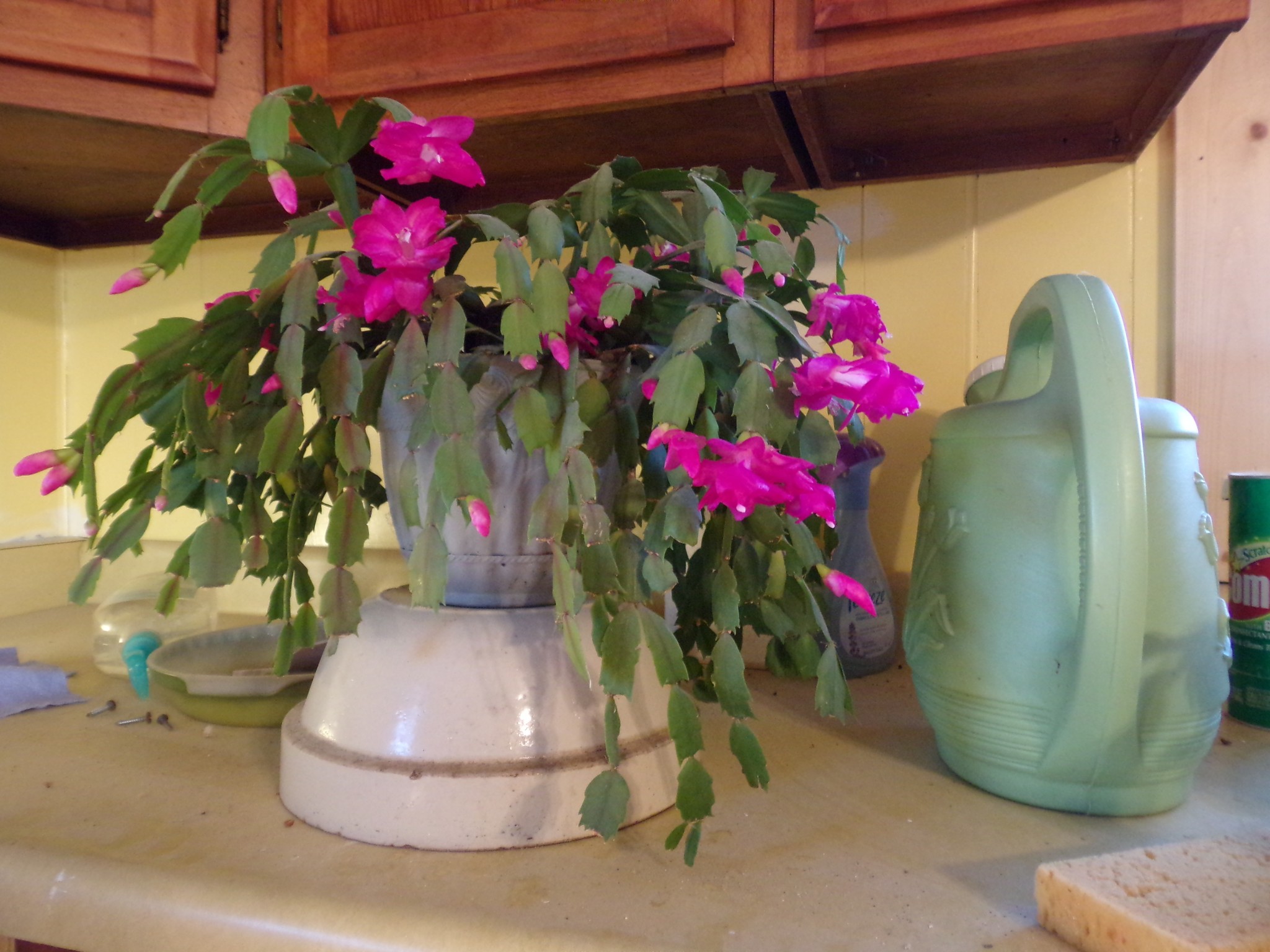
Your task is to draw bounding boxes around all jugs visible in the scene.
[900,271,1236,815]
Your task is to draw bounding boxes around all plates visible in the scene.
[145,620,326,729]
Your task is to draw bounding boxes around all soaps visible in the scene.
[286,639,329,674]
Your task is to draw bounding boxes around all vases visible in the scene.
[381,354,624,605]
[818,434,898,679]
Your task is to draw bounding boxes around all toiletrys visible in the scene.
[90,569,222,701]
[817,432,901,681]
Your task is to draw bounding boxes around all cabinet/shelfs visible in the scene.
[273,0,1250,217]
[0,0,284,250]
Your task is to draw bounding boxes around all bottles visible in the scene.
[801,433,904,675]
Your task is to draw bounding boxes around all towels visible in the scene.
[0,646,90,719]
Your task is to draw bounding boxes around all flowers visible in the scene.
[12,85,924,868]
